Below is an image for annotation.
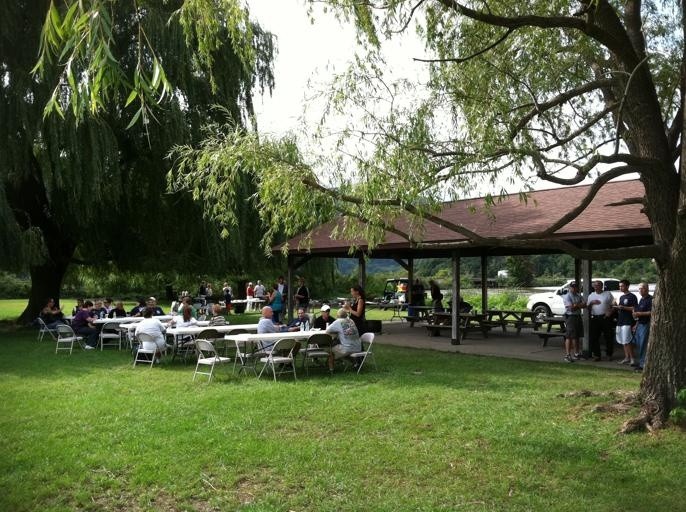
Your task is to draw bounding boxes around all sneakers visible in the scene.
[592,356,602,362]
[621,359,629,364]
[574,352,586,361]
[630,359,636,365]
[564,354,573,363]
[84,344,95,349]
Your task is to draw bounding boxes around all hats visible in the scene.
[570,281,578,287]
[321,304,330,313]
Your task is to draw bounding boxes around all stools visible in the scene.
[532,331,566,347]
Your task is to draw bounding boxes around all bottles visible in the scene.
[100,310,105,320]
[170,313,176,329]
[305,320,309,333]
[300,322,304,333]
[113,311,116,318]
[71,308,75,316]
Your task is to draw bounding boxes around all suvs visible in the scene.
[384,277,427,311]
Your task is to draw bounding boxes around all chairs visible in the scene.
[233,333,268,377]
[258,338,297,382]
[298,334,333,376]
[403,306,542,340]
[36,314,281,369]
[344,332,378,374]
[192,339,231,386]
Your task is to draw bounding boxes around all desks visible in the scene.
[219,300,267,313]
[224,329,336,377]
[378,303,409,324]
[543,317,566,332]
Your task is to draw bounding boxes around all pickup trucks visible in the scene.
[527,278,655,324]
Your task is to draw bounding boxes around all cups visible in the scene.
[633,310,639,321]
[105,314,108,319]
[472,310,477,315]
[326,323,330,331]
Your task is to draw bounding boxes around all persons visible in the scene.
[393,279,473,326]
[562,280,587,362]
[41,296,166,364]
[613,279,638,366]
[632,282,653,370]
[170,277,368,372]
[586,280,617,361]
